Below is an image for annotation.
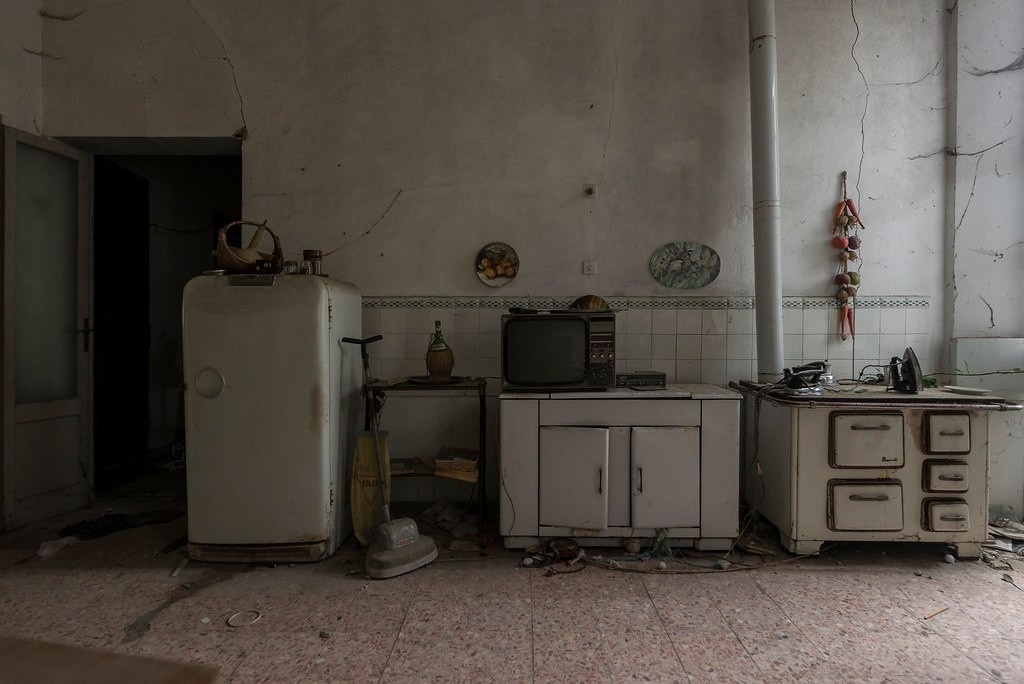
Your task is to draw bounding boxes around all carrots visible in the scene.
[832,199,865,234]
[840,306,854,339]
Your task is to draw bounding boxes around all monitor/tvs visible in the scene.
[502,312,617,392]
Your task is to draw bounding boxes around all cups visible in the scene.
[303,249,321,274]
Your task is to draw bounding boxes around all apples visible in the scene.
[832,235,849,249]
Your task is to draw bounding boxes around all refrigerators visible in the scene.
[180,274,363,562]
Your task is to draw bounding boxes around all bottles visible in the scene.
[426,321,454,383]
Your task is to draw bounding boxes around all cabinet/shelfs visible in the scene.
[361,375,489,549]
[497,383,742,550]
[741,380,990,558]
